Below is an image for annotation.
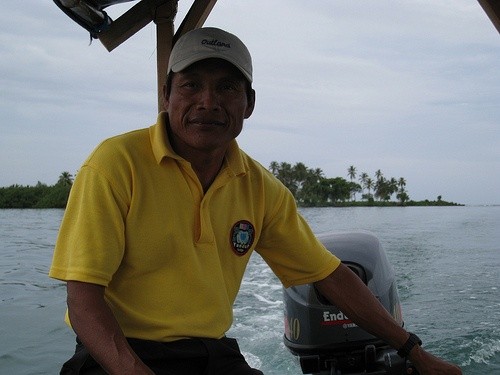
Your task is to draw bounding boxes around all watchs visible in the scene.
[398,332,423,357]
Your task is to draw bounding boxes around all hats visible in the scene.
[167,26,252,84]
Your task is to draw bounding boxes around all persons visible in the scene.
[48,27,463,375]
